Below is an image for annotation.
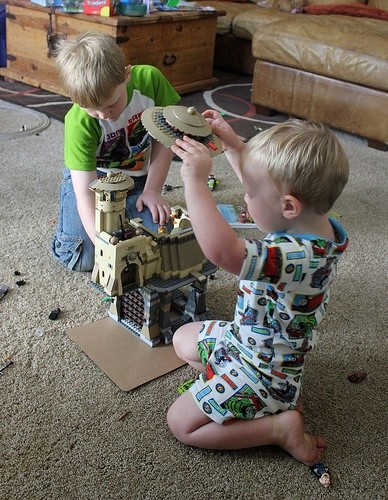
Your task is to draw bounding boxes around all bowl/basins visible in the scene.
[122,4,148,17]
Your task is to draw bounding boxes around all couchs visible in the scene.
[251,14,388,152]
[187,0,388,76]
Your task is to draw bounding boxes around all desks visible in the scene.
[0,0,227,98]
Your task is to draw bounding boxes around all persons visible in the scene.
[311,464,332,488]
[50,31,181,272]
[167,109,349,467]
